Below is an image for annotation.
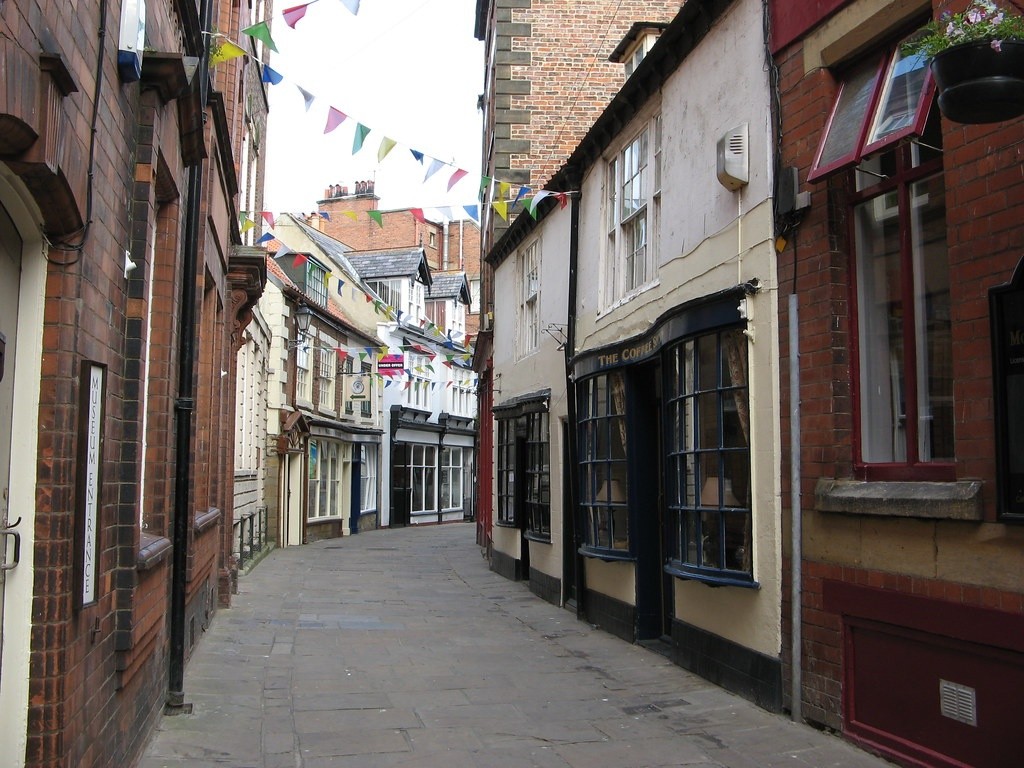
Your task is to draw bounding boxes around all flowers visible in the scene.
[900,0,1024,61]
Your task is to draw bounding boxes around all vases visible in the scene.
[930,35,1024,123]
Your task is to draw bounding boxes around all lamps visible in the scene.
[700,477,741,507]
[595,480,626,548]
[288,302,314,347]
